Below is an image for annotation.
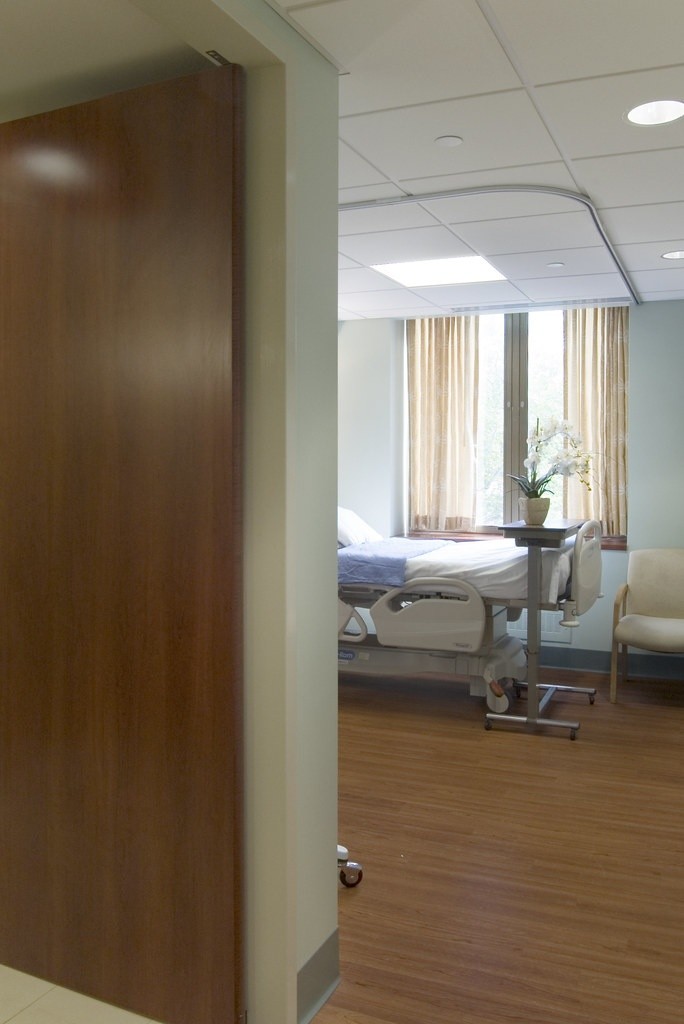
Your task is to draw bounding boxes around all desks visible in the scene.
[484,517,597,741]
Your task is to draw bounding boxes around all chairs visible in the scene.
[608,548,684,704]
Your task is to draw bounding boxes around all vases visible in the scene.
[519,497,551,525]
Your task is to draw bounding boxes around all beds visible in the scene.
[337,505,604,716]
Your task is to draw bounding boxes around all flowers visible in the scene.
[522,408,600,494]
